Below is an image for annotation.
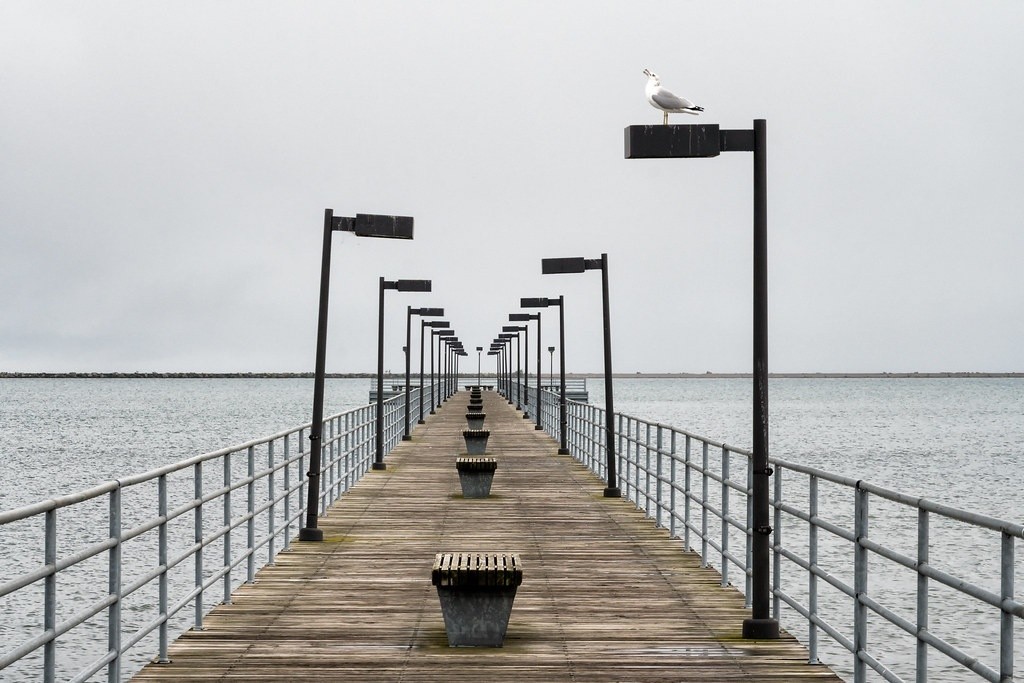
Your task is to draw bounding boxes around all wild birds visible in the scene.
[640,70,704,124]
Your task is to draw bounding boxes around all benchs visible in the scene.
[431,553,523,650]
[465,412,486,429]
[462,429,490,454]
[455,457,497,497]
[465,385,494,412]
